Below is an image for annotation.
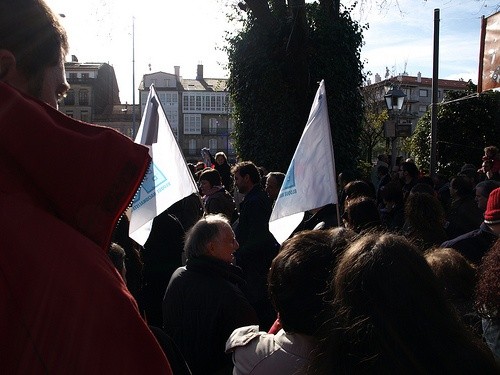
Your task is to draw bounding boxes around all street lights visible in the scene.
[383,81,407,168]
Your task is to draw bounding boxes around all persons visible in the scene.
[104,145,214,326]
[215,146,500,374]
[163,214,262,375]
[0,0,173,375]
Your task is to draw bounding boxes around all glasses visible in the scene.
[212,237,237,248]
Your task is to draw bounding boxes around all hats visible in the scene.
[484,188,500,224]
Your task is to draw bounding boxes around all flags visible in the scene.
[268,78,338,246]
[125,84,198,247]
